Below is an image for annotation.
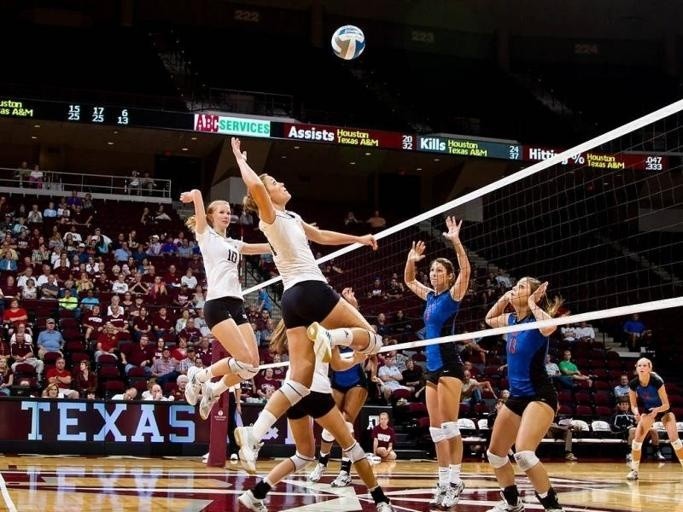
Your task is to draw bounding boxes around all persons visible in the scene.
[240,288,287,398]
[576,322,595,344]
[544,406,578,461]
[624,313,647,348]
[401,360,425,397]
[561,324,576,344]
[142,172,156,197]
[485,276,563,512]
[372,267,403,347]
[179,189,271,420]
[202,335,214,367]
[614,375,631,402]
[230,137,382,473]
[611,397,665,460]
[1,189,203,400]
[459,269,489,412]
[559,351,599,388]
[404,217,471,509]
[376,350,416,405]
[309,345,368,486]
[238,345,393,512]
[546,355,578,387]
[125,170,140,195]
[626,358,683,479]
[372,412,397,460]
[14,160,43,189]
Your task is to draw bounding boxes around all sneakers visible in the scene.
[310,463,328,482]
[184,365,207,406]
[374,500,397,512]
[535,488,564,512]
[230,454,238,460]
[233,426,264,475]
[626,469,639,480]
[429,481,449,508]
[441,481,466,509]
[306,321,334,363]
[486,485,525,512]
[238,488,268,512]
[199,381,221,421]
[330,471,353,488]
[655,452,665,461]
[565,452,577,461]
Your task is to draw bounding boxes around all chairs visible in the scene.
[459,403,468,415]
[36,300,57,328]
[60,317,79,339]
[590,351,603,368]
[577,352,587,367]
[478,419,489,440]
[390,389,426,422]
[610,371,622,387]
[557,418,579,443]
[677,421,683,444]
[11,363,37,389]
[478,376,497,398]
[593,368,609,388]
[540,422,556,443]
[558,389,574,414]
[649,422,664,444]
[415,415,432,444]
[592,420,622,444]
[485,366,497,375]
[607,351,620,367]
[501,379,509,387]
[665,421,674,443]
[67,340,89,361]
[24,299,36,321]
[669,394,683,422]
[571,419,601,443]
[470,367,479,377]
[593,389,612,417]
[102,379,125,391]
[412,353,426,361]
[457,417,481,442]
[666,383,681,393]
[95,355,122,378]
[576,390,592,415]
[127,367,146,391]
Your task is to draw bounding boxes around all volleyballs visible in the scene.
[330,25,366,60]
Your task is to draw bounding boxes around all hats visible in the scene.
[46,318,55,324]
[150,234,159,239]
[187,347,194,352]
